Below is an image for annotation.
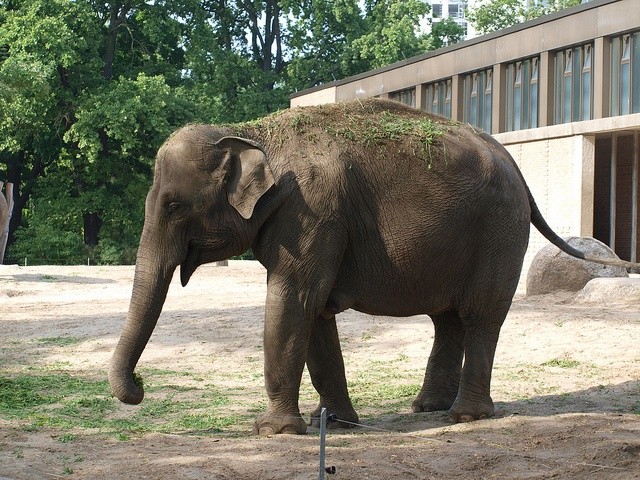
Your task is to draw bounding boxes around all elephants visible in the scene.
[106,95,640,437]
